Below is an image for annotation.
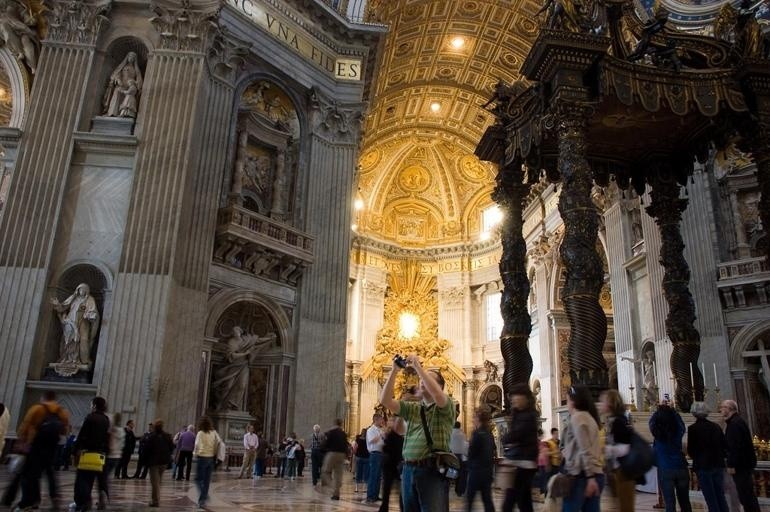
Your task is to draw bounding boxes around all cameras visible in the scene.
[392,354,407,368]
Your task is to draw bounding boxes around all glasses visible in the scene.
[719,405,731,409]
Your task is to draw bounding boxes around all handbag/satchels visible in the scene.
[546,474,572,499]
[418,405,460,479]
[619,420,651,481]
[216,430,227,466]
[76,448,106,473]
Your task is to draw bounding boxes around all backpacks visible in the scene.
[32,401,65,456]
[214,432,227,465]
[612,414,654,486]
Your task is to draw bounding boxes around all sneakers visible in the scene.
[15,500,40,511]
[70,501,80,509]
[291,477,295,481]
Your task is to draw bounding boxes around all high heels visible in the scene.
[354,489,358,493]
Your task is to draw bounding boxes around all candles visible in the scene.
[701,362,708,388]
[628,361,632,386]
[652,360,659,388]
[640,361,646,387]
[689,361,695,388]
[712,362,719,390]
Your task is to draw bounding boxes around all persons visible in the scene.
[720,400,758,512]
[621,350,657,408]
[192,414,220,507]
[255,431,270,477]
[352,429,370,492]
[448,421,469,497]
[286,441,301,479]
[276,438,289,479]
[50,284,99,378]
[147,420,175,507]
[687,404,729,511]
[118,78,139,119]
[546,427,563,472]
[172,425,188,478]
[176,427,196,483]
[107,412,124,475]
[648,400,691,511]
[18,391,70,508]
[383,354,455,511]
[502,385,538,511]
[380,391,414,510]
[535,430,551,495]
[70,397,110,510]
[597,391,638,511]
[323,421,347,499]
[238,427,258,478]
[310,424,328,484]
[564,387,606,512]
[214,327,275,412]
[102,50,146,119]
[116,420,135,478]
[297,440,306,477]
[366,415,386,501]
[467,412,496,511]
[132,424,159,479]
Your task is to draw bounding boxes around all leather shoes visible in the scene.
[366,495,384,502]
[235,476,243,479]
[457,492,461,496]
[652,502,667,509]
[122,476,130,480]
[62,466,69,472]
[197,503,206,507]
[298,474,304,477]
[149,501,159,506]
[186,477,190,480]
[176,477,183,481]
[130,475,140,479]
[331,496,339,500]
[139,476,146,479]
[246,476,254,479]
[113,474,121,480]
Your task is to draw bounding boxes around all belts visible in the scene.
[401,460,427,466]
